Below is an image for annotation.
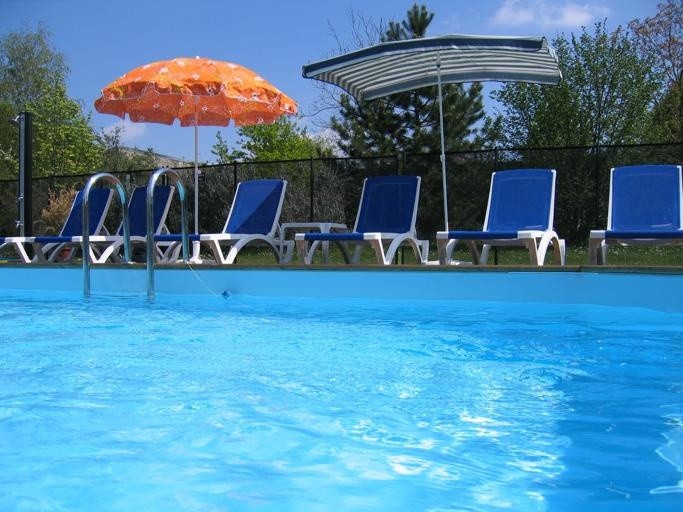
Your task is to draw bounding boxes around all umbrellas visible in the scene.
[94,56,299,235]
[302,34,563,231]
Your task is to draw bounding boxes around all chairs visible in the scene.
[294,175,429,264]
[30,185,177,265]
[587,165,681,266]
[436,170,565,267]
[1,187,112,266]
[147,179,296,266]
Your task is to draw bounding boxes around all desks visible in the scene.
[278,222,346,263]
